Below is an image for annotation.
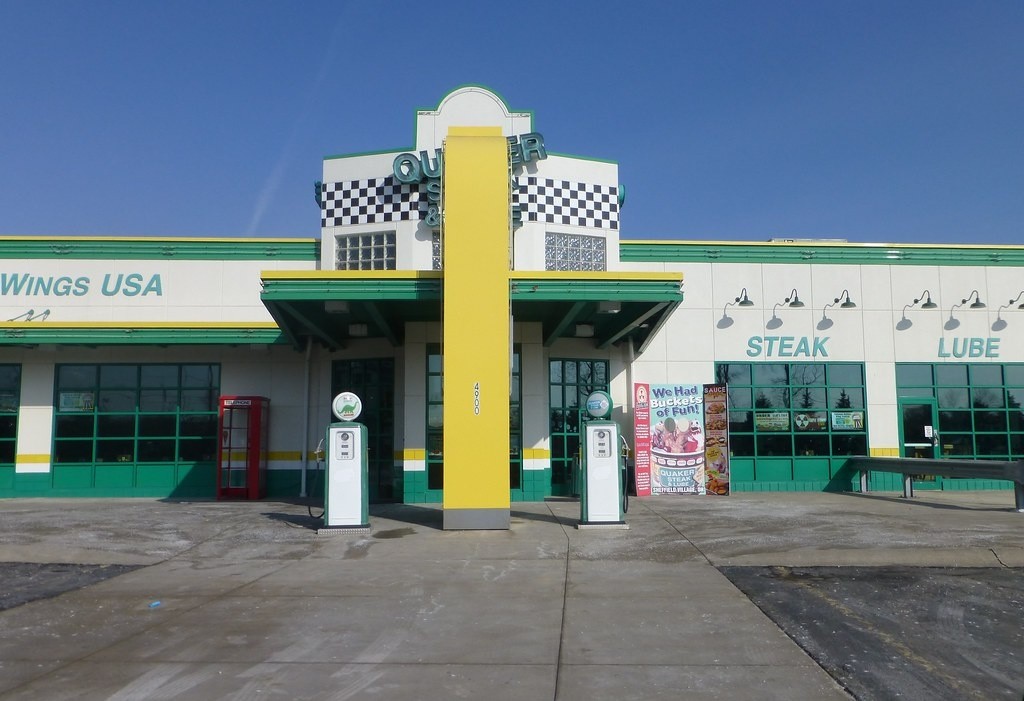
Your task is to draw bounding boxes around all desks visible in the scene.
[915,444,954,481]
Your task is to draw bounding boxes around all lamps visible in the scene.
[962,290,987,309]
[915,290,938,309]
[736,287,754,307]
[1010,291,1024,309]
[785,288,806,307]
[835,289,856,308]
